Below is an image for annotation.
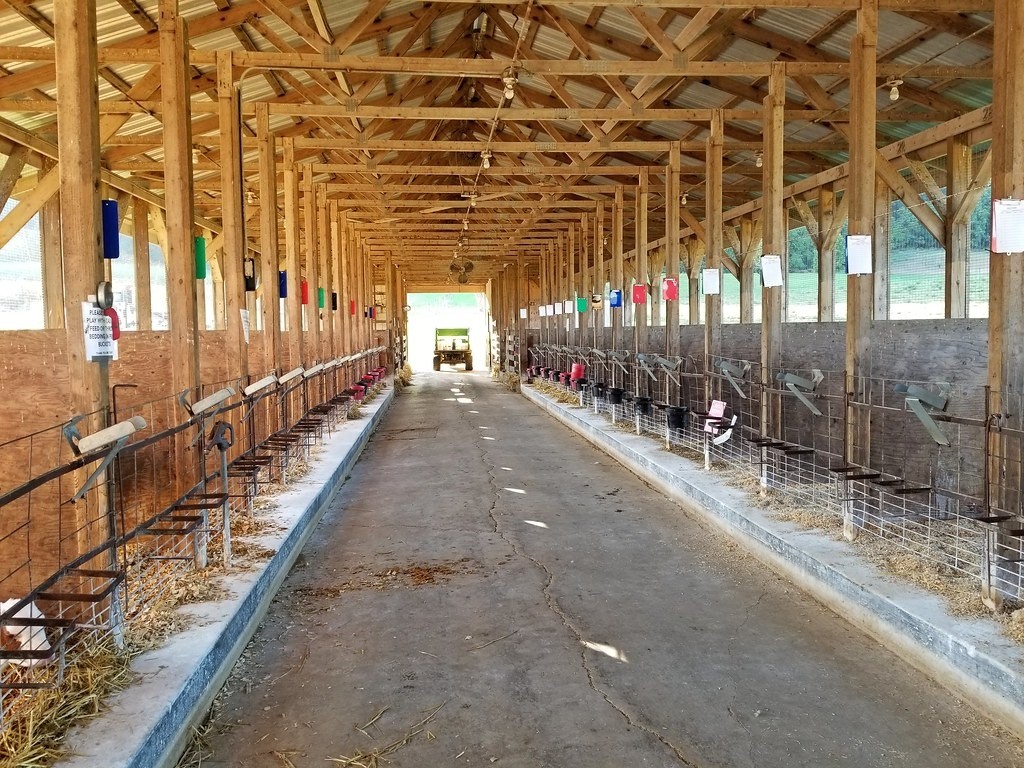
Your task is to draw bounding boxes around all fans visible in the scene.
[403,305,411,312]
[448,257,476,286]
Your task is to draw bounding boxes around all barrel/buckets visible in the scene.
[606,388,623,404]
[634,397,652,415]
[553,370,561,382]
[362,375,373,387]
[593,383,604,397]
[564,375,570,386]
[528,378,533,384]
[665,406,688,428]
[571,364,584,389]
[544,368,551,378]
[549,371,553,380]
[560,372,569,384]
[378,366,386,374]
[540,368,544,377]
[576,378,586,391]
[376,368,384,379]
[527,369,531,377]
[532,366,535,375]
[530,370,536,377]
[368,371,378,382]
[352,386,364,400]
[535,367,541,375]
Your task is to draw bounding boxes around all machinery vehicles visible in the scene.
[433,328,473,371]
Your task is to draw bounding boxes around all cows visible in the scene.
[0,596,55,675]
